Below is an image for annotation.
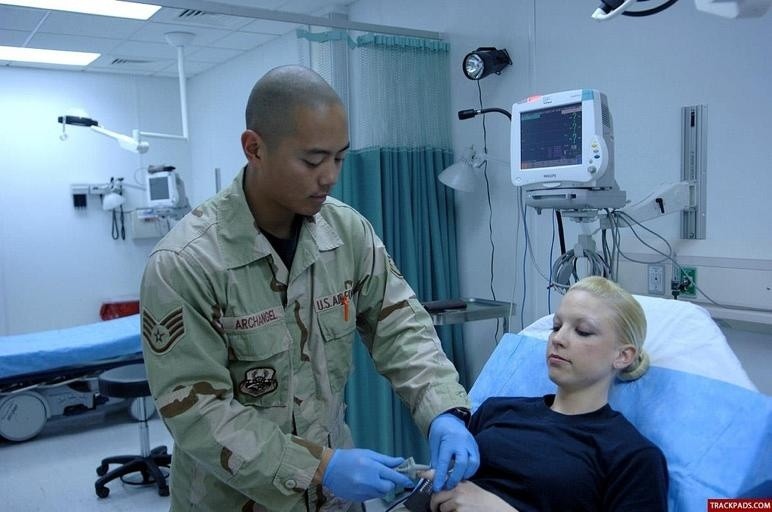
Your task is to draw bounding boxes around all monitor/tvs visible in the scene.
[145,171,188,208]
[509,89,621,190]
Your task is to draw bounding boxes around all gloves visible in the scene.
[429,413,480,492]
[322,448,414,502]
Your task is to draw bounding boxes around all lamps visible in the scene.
[57,31,197,155]
[463,48,511,81]
[104,179,145,210]
[438,146,510,194]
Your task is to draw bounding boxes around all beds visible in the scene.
[419,292,772,512]
[0,313,142,443]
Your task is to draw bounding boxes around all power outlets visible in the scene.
[649,264,664,294]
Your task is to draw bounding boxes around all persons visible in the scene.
[136,61,482,511]
[415,274,669,511]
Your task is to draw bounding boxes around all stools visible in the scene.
[96,364,171,498]
